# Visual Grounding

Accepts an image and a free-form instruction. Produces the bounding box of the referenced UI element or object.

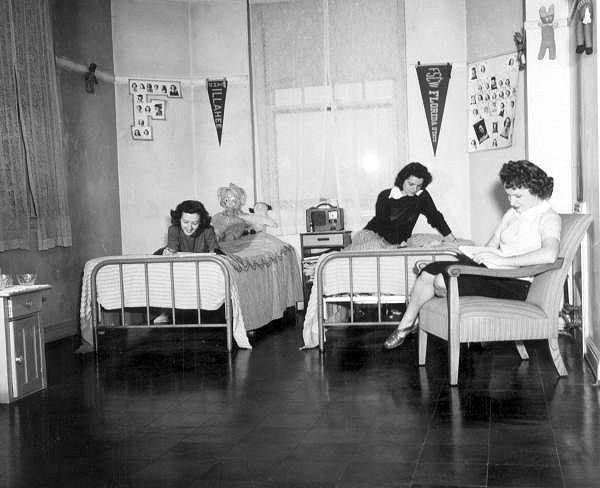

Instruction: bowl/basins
[0,272,38,292]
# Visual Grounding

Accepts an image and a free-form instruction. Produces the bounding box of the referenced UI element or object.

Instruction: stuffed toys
[210,183,245,241]
[243,202,277,235]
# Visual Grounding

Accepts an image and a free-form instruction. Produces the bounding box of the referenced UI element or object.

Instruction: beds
[72,232,299,354]
[299,231,477,354]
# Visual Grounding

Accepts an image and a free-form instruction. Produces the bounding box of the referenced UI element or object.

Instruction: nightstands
[300,230,353,306]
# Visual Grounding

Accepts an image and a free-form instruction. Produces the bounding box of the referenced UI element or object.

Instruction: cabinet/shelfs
[1,281,53,404]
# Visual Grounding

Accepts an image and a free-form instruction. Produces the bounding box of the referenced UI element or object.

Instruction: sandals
[384,315,420,351]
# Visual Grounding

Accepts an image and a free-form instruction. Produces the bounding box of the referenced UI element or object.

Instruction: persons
[170,85,179,96]
[477,125,488,143]
[162,201,220,253]
[571,0,593,54]
[346,163,456,251]
[383,159,561,350]
[537,5,556,61]
[501,118,510,138]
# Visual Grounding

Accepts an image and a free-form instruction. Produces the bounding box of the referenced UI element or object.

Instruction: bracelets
[453,238,456,243]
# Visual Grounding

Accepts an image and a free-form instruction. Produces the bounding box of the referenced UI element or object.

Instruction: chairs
[406,212,591,388]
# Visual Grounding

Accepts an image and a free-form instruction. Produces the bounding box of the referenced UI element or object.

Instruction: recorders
[305,202,344,232]
